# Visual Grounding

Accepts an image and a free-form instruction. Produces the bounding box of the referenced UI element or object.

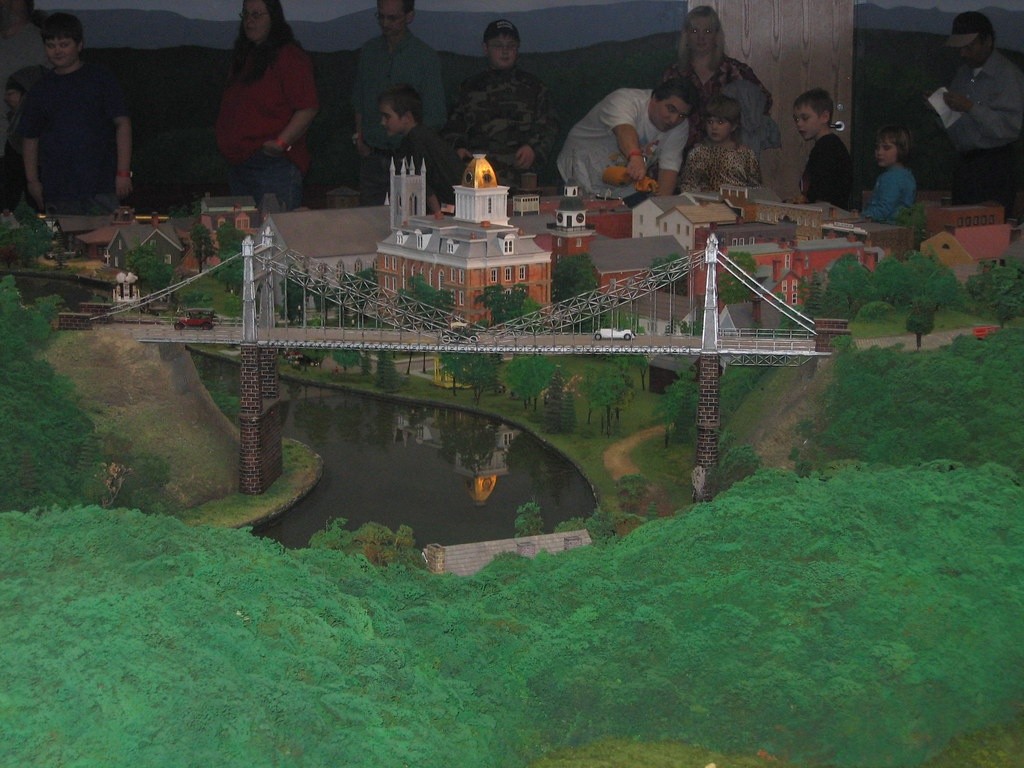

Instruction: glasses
[375,12,407,22]
[488,43,519,51]
[239,11,269,19]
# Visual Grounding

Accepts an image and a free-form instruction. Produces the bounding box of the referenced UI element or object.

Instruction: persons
[212,0,321,214]
[924,12,1024,224]
[0,0,153,216]
[352,0,915,224]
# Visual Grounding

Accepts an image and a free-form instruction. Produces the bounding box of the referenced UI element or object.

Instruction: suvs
[174,308,215,331]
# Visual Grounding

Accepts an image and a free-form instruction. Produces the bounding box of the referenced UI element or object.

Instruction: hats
[946,11,991,46]
[484,20,520,42]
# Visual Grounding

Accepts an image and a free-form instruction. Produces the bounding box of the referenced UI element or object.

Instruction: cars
[441,327,480,343]
[594,328,635,341]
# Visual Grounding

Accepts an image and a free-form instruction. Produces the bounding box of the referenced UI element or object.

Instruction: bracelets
[117,169,133,178]
[628,150,648,164]
[355,131,363,140]
[273,136,292,152]
